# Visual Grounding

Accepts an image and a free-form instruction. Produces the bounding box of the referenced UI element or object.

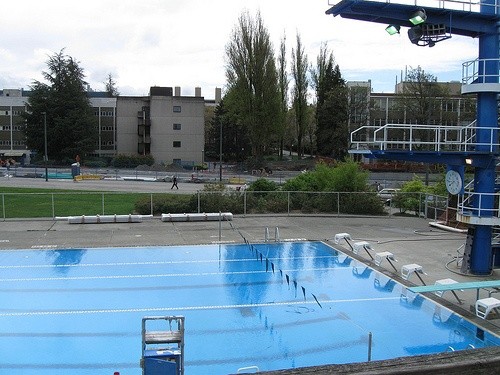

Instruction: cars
[422,195,447,215]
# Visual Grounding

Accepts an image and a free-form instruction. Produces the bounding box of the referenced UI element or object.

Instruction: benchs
[162,212,233,222]
[67,215,142,222]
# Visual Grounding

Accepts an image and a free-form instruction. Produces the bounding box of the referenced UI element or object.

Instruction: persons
[171,176,178,190]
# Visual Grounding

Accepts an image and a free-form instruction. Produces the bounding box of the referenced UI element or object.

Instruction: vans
[375,188,403,206]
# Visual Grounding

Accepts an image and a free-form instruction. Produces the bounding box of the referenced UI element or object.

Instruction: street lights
[39,112,49,182]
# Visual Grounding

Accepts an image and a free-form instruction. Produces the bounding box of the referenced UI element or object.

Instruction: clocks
[445,170,462,195]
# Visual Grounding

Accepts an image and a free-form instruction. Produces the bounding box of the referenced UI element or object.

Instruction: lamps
[385,24,400,35]
[427,37,436,47]
[407,27,423,45]
[465,157,473,164]
[408,8,427,25]
